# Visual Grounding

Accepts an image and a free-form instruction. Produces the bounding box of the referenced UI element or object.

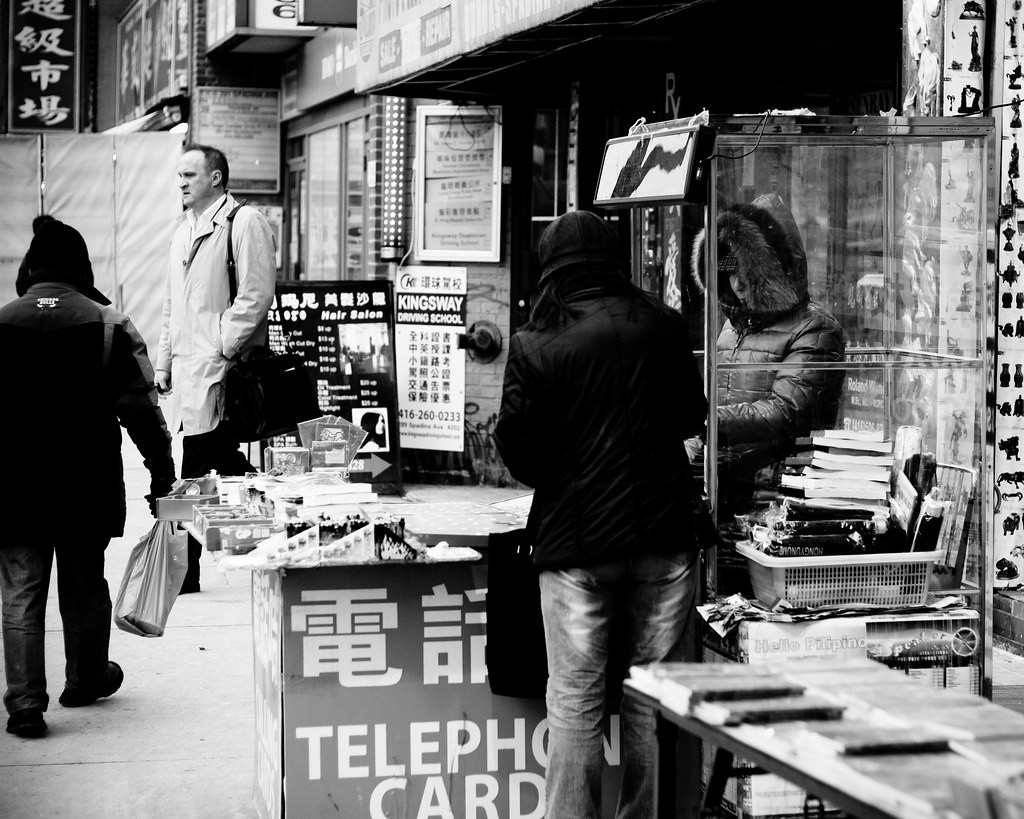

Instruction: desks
[623,678,930,818]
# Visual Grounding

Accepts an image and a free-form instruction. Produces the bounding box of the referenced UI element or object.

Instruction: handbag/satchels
[113,519,187,639]
[219,345,318,445]
[487,489,641,714]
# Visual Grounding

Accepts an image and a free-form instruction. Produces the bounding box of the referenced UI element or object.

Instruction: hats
[16,214,112,307]
[537,209,624,286]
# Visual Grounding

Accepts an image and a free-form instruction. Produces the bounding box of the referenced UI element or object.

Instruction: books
[764,429,895,553]
[629,654,1022,819]
[293,481,383,519]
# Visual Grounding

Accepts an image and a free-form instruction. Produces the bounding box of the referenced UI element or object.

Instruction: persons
[490,209,722,819]
[684,190,849,819]
[153,144,277,596]
[0,212,180,738]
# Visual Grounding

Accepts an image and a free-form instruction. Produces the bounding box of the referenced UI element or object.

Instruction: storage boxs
[193,505,273,552]
[156,494,220,520]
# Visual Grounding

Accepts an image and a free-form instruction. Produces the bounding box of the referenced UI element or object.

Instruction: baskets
[735,539,947,609]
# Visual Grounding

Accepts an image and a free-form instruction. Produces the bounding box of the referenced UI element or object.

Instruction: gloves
[142,480,174,519]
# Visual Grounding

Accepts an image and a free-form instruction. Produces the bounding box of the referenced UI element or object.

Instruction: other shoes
[6,714,51,738]
[59,661,124,708]
